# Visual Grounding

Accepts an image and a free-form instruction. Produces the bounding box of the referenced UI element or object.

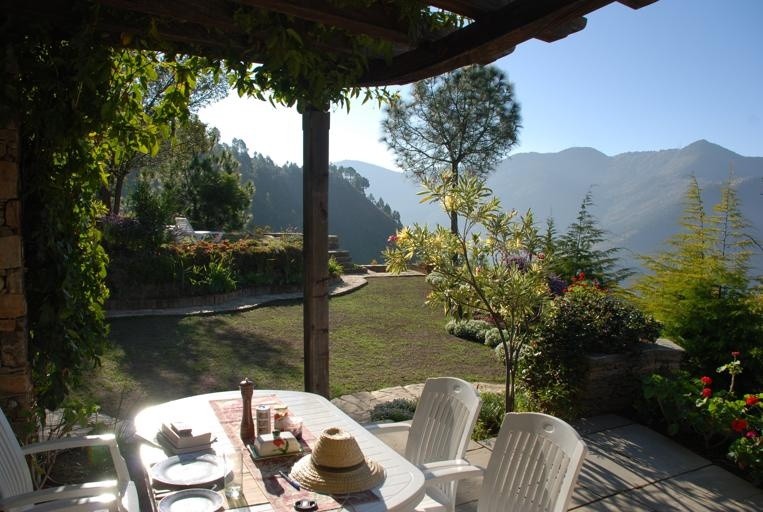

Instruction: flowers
[694,350,763,473]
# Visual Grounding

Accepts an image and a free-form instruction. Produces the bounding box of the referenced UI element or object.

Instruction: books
[153,422,211,455]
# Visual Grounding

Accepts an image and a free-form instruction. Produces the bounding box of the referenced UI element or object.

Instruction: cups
[223,449,244,498]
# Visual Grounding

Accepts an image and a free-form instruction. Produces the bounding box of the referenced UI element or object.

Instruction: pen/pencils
[279,470,299,489]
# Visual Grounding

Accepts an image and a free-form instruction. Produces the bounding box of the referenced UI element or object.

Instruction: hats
[290,427,387,495]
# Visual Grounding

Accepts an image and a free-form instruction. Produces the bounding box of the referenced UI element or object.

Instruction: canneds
[256,405,270,435]
[274,405,288,431]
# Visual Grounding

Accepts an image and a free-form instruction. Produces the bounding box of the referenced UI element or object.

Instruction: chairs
[362,375,588,512]
[0,408,141,511]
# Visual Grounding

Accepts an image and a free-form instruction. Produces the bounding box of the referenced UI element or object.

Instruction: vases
[707,430,740,475]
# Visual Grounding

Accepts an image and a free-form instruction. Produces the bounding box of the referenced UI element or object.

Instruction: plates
[159,488,224,512]
[247,438,311,460]
[150,452,233,486]
